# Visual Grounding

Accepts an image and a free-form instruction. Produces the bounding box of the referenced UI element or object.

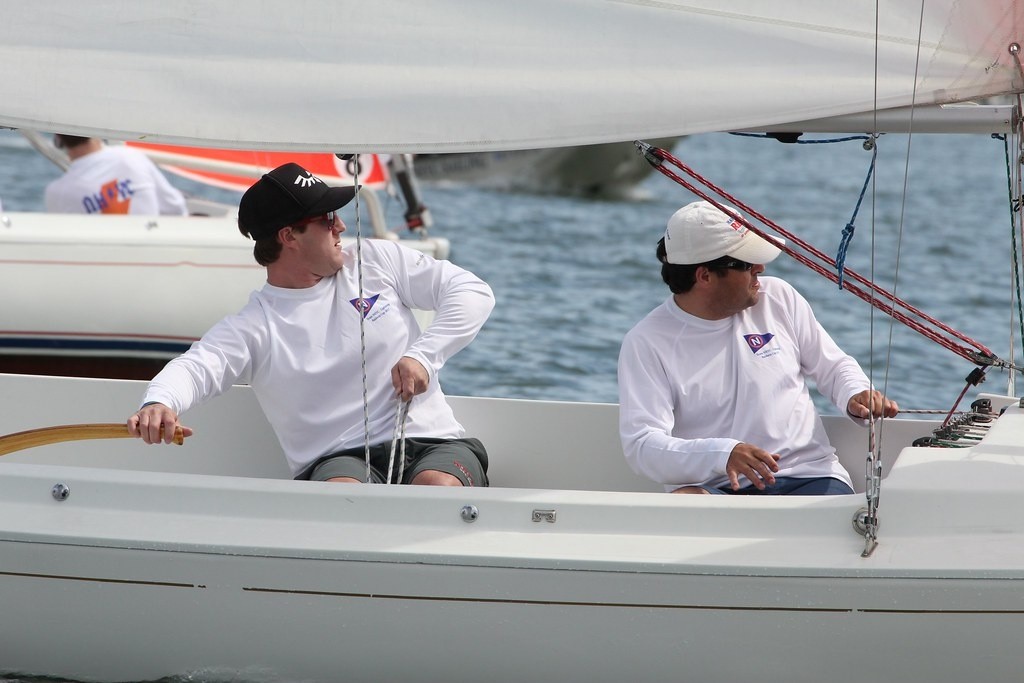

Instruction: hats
[238,163,362,240]
[661,200,785,264]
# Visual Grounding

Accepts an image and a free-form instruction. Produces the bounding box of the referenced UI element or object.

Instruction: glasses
[706,259,753,270]
[286,211,335,227]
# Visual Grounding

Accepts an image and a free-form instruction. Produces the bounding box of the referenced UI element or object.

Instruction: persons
[46,133,187,216]
[618,200,898,496]
[126,162,496,486]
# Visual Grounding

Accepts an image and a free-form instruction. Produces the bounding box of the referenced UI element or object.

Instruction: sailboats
[0,123,453,381]
[0,0,1024,683]
